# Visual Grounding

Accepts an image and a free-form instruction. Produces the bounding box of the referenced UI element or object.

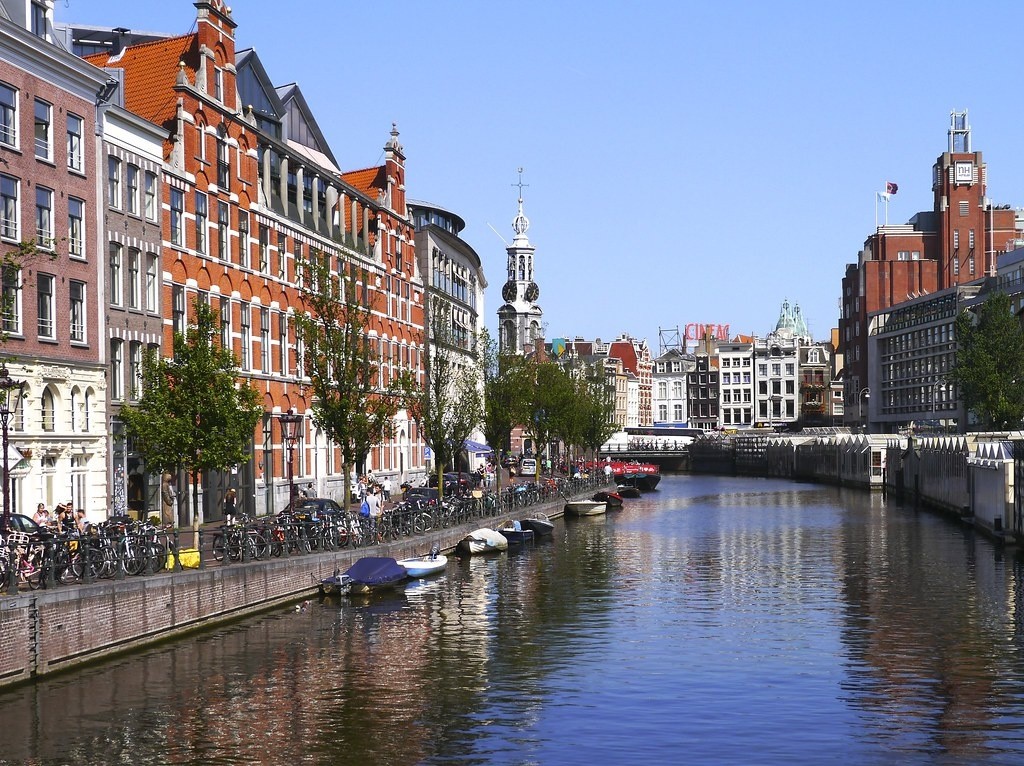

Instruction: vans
[518,458,537,476]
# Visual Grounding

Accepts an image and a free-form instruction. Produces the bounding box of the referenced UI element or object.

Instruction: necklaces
[37,511,42,517]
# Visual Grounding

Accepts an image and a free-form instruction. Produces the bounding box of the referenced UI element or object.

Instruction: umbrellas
[914,419,942,434]
[444,438,495,457]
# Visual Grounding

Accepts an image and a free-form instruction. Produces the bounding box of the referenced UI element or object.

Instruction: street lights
[932,379,948,421]
[278,410,304,511]
[858,386,871,433]
[0,369,25,544]
[639,398,651,426]
[551,440,559,477]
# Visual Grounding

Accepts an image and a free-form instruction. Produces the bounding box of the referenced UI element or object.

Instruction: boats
[454,527,509,556]
[617,485,642,498]
[560,491,608,517]
[495,518,534,544]
[395,542,449,579]
[592,491,623,507]
[318,553,408,597]
[519,512,554,535]
[611,459,662,491]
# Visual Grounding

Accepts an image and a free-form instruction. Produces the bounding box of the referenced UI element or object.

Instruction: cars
[501,456,517,467]
[278,495,344,523]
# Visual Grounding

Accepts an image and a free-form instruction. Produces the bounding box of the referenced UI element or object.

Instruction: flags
[877,181,898,203]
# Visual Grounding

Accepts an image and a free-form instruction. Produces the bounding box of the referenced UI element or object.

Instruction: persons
[373,484,384,540]
[224,488,239,526]
[476,448,613,518]
[360,488,381,546]
[32,502,88,560]
[358,469,392,504]
[377,190,387,207]
[293,481,316,500]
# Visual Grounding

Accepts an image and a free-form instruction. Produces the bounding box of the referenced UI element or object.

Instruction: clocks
[502,281,518,303]
[527,283,540,301]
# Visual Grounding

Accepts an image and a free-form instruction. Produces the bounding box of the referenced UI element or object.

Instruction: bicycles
[210,464,606,564]
[0,509,178,592]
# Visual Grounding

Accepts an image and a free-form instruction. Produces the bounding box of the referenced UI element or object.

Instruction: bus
[622,425,717,451]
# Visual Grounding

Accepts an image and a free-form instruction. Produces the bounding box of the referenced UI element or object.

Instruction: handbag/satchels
[360,497,370,518]
